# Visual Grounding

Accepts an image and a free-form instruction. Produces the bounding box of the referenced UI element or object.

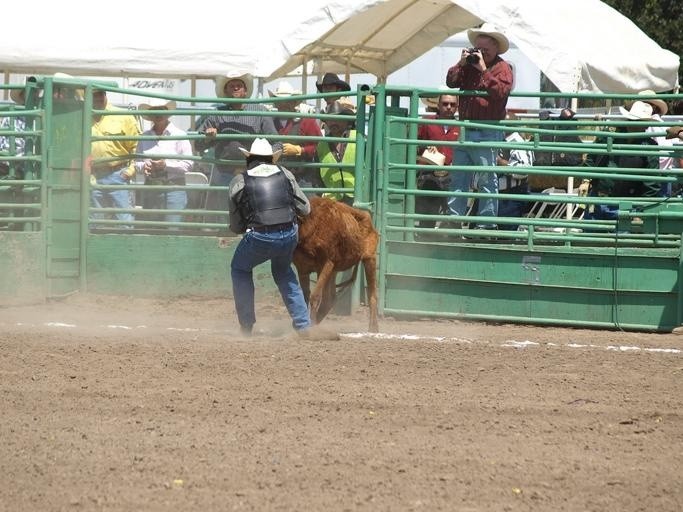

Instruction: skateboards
[291,196,379,333]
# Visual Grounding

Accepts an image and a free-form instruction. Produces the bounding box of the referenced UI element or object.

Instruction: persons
[89,88,139,228]
[440,26,513,244]
[265,83,322,183]
[416,93,535,240]
[226,136,310,338]
[314,74,359,204]
[133,100,194,230]
[193,77,284,236]
[578,90,681,233]
[1,88,42,179]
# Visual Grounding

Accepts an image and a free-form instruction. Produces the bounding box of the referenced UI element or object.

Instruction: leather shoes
[666,126,682,139]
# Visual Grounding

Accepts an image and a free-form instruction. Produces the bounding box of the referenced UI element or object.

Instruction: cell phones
[465,48,482,65]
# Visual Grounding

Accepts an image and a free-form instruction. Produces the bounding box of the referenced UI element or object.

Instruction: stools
[465,127,482,132]
[95,164,128,176]
[257,225,294,232]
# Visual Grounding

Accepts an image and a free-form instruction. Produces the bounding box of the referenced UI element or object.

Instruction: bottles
[638,89,669,116]
[319,100,356,124]
[421,96,441,108]
[215,71,254,98]
[267,82,302,105]
[137,95,176,119]
[619,101,665,121]
[9,88,26,104]
[415,149,445,166]
[315,73,352,92]
[466,22,509,54]
[239,137,283,163]
